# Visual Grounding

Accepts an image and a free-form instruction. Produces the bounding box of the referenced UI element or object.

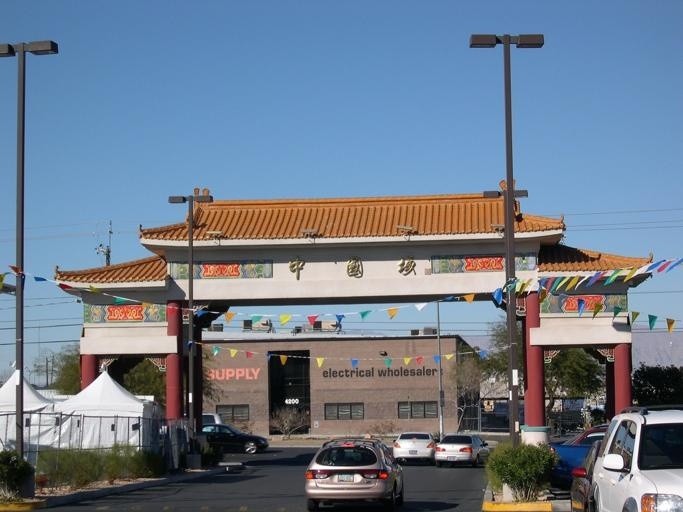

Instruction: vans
[159,414,237,435]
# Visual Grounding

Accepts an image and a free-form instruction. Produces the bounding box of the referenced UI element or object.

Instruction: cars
[435,434,490,467]
[304,439,404,511]
[547,409,683,512]
[393,432,435,465]
[201,426,268,453]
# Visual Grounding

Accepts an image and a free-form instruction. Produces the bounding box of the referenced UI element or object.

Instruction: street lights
[470,36,544,449]
[168,196,211,470]
[1,41,58,499]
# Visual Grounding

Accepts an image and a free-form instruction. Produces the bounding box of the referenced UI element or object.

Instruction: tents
[0,369,156,457]
